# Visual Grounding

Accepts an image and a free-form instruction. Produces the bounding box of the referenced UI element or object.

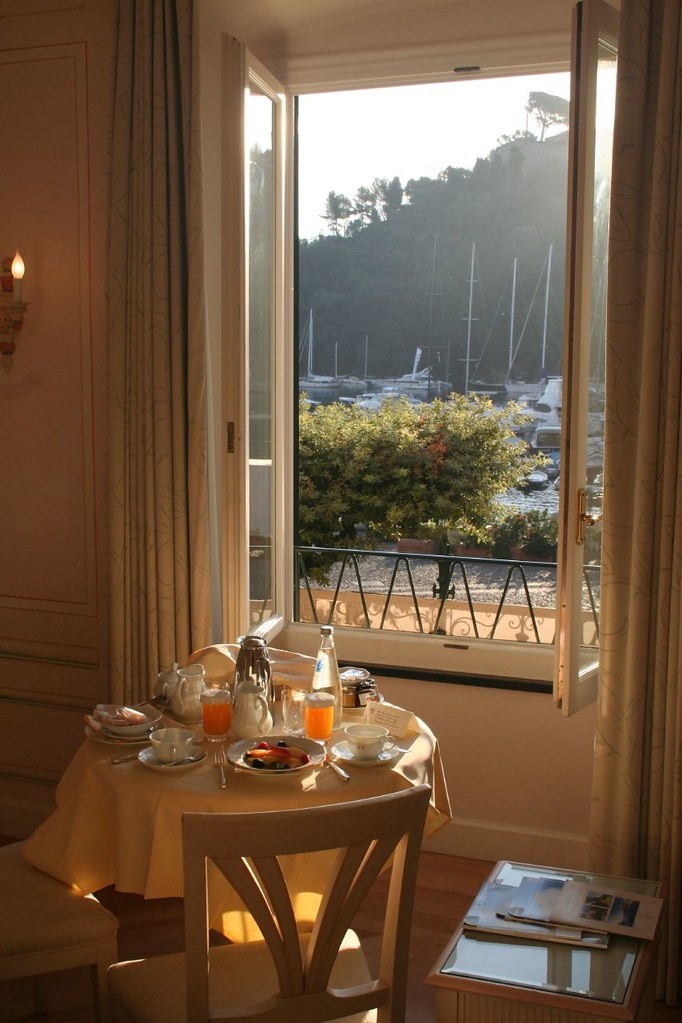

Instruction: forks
[213,746,229,789]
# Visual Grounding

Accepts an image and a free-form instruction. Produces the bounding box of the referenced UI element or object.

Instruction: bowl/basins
[102,705,163,736]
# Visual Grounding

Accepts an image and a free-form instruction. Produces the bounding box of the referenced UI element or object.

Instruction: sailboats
[303,237,561,497]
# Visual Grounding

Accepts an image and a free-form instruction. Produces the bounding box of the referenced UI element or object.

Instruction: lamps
[0,245,31,372]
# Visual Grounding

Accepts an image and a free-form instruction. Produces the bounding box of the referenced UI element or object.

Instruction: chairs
[105,785,433,1023]
[0,839,120,1022]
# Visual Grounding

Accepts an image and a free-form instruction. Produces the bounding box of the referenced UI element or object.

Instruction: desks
[424,861,664,1023]
[20,696,452,944]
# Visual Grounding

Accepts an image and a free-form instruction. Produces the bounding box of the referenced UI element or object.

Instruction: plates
[226,734,327,776]
[331,740,400,766]
[137,746,208,774]
[84,721,167,745]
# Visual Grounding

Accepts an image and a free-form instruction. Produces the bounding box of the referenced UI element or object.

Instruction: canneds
[341,678,380,709]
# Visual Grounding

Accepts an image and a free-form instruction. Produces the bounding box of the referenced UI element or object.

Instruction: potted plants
[399,499,558,561]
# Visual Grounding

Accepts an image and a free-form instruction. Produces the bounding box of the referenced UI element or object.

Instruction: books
[463,876,663,950]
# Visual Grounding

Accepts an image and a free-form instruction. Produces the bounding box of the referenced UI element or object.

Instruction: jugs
[170,663,208,724]
[154,663,182,706]
[229,682,273,738]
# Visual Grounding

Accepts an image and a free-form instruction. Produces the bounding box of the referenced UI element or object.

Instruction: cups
[149,728,195,763]
[280,688,308,736]
[343,724,396,759]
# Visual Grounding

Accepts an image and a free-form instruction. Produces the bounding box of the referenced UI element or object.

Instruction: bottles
[310,625,343,730]
[305,692,336,741]
[200,688,233,743]
